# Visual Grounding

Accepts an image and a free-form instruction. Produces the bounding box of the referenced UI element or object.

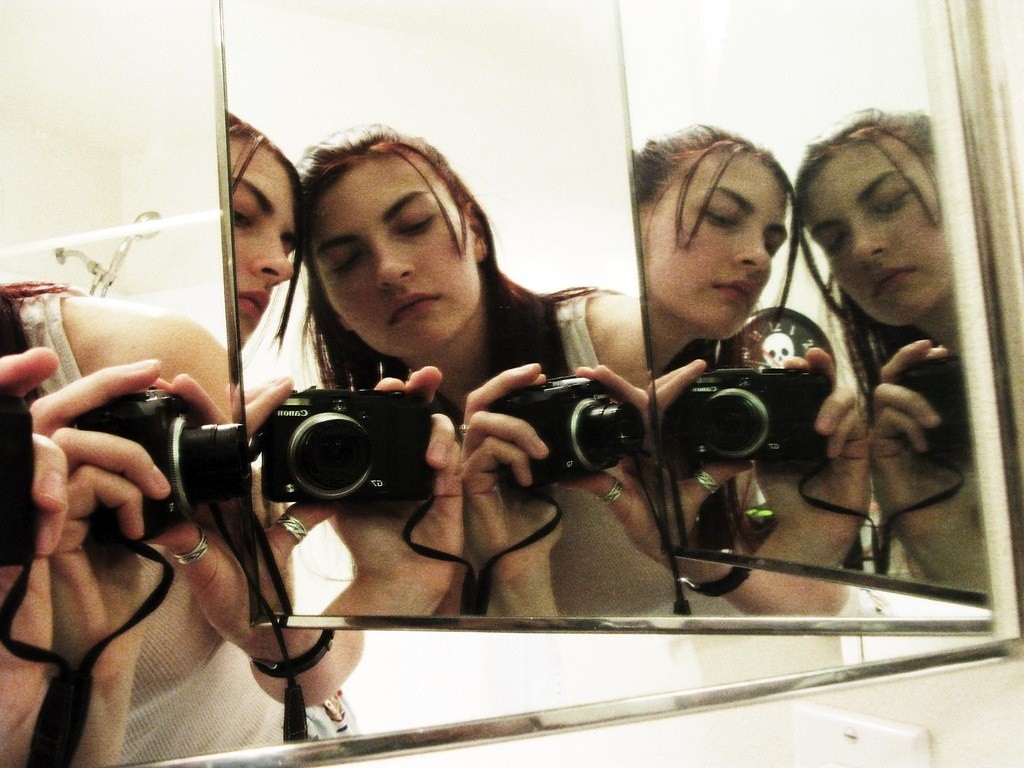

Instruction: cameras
[71,391,253,557]
[666,366,832,465]
[0,394,38,566]
[262,392,438,510]
[488,375,645,499]
[897,356,973,457]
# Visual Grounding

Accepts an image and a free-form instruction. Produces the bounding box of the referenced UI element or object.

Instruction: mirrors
[208,0,1007,636]
[122,44,1021,768]
[616,0,997,609]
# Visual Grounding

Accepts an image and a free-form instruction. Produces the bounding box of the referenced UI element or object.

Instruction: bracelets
[678,567,751,598]
[249,629,334,679]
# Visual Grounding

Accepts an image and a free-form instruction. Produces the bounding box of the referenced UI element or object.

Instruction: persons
[0,282,364,768]
[228,112,850,615]
[631,113,990,592]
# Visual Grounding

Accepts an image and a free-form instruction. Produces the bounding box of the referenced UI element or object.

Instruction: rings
[694,468,717,493]
[599,477,624,503]
[169,524,210,565]
[278,514,308,544]
